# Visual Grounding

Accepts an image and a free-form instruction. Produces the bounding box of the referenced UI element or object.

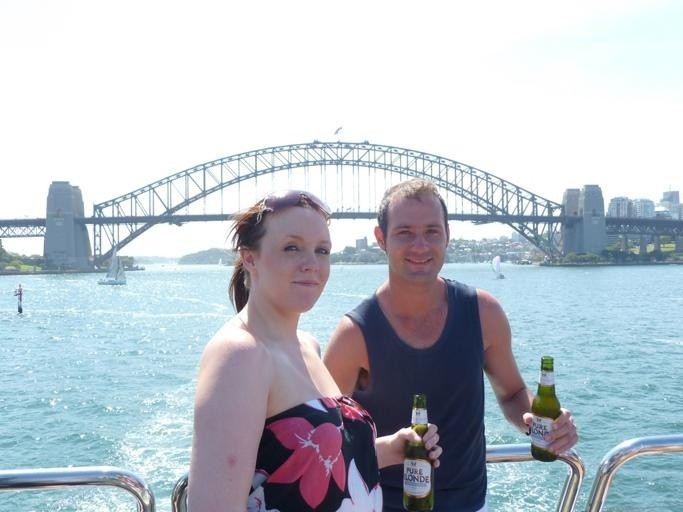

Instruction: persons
[187,190,444,512]
[323,179,579,511]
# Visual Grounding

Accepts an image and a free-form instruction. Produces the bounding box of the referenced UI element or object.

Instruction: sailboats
[97,246,127,285]
[492,255,505,279]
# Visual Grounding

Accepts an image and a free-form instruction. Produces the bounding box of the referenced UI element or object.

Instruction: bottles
[402,392,434,511]
[531,355,561,462]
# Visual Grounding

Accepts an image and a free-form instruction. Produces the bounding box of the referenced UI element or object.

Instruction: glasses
[264,188,332,215]
